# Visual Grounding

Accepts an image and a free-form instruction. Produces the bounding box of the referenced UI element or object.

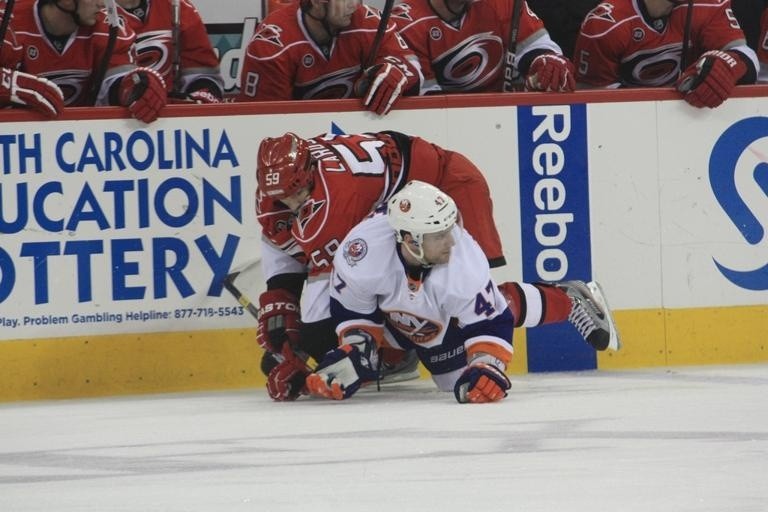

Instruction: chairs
[260,351,285,377]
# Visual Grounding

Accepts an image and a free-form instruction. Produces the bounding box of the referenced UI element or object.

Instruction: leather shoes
[522,51,576,94]
[305,343,368,400]
[351,54,420,116]
[674,49,750,109]
[117,68,167,125]
[265,352,319,403]
[452,353,511,403]
[254,289,300,356]
[0,67,65,119]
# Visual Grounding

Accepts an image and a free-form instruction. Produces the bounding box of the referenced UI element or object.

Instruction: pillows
[387,179,457,244]
[256,132,317,203]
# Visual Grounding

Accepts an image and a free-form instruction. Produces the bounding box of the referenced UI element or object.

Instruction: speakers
[532,284,610,353]
[368,347,419,379]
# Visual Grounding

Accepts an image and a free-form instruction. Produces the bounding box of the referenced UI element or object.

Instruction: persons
[573,1,767,108]
[1,0,225,121]
[262,178,515,405]
[227,1,576,116]
[253,129,610,400]
